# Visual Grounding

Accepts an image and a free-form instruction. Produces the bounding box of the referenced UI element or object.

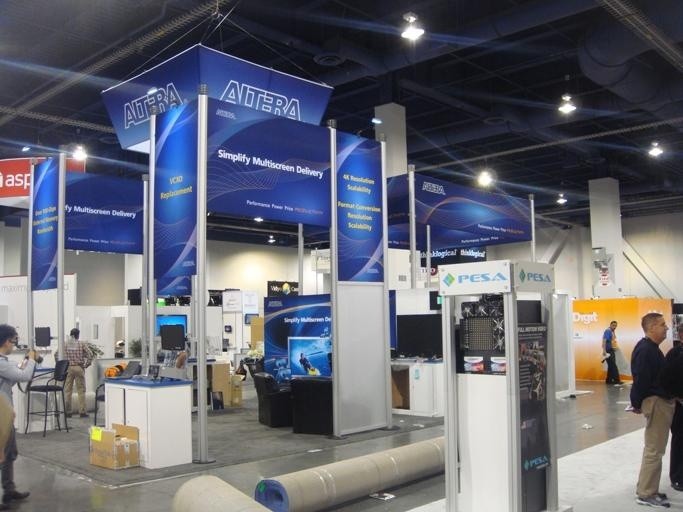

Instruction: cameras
[25,349,43,364]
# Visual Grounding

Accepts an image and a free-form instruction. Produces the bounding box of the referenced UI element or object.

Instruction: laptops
[107,361,140,380]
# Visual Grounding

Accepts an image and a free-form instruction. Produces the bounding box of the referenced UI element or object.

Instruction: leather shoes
[671,482,683,491]
[2,492,30,503]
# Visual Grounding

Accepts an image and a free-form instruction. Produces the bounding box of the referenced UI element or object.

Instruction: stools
[92,361,141,425]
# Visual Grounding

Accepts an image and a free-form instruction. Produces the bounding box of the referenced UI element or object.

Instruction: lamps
[591,245,610,273]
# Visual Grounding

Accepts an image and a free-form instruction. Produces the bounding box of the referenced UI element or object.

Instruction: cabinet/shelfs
[389,359,444,418]
[189,363,229,412]
[101,377,195,471]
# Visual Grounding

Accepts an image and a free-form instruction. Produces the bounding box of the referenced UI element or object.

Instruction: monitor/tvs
[160,324,185,350]
[35,327,50,346]
[156,314,187,336]
[245,314,259,324]
[287,336,332,377]
[397,314,442,362]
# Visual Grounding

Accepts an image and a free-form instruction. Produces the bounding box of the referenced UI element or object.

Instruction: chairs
[290,378,332,434]
[252,371,293,428]
[24,360,73,436]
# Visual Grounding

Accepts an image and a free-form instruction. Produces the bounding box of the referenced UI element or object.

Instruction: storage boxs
[230,373,245,406]
[89,421,141,470]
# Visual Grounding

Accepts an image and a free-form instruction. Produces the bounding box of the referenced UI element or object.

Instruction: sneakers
[635,492,670,509]
[80,413,89,417]
[67,414,72,418]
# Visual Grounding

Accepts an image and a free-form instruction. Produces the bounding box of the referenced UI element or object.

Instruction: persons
[299,353,315,374]
[631,312,676,509]
[602,321,624,385]
[0,325,39,508]
[666,322,683,490]
[55,328,93,417]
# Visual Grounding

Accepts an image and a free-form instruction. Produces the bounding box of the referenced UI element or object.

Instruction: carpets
[6,378,444,488]
